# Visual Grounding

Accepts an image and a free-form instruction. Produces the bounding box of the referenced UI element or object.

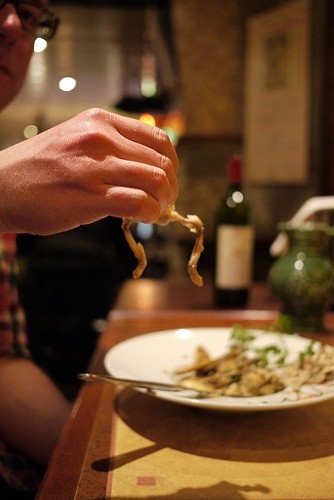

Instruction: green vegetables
[228,324,314,370]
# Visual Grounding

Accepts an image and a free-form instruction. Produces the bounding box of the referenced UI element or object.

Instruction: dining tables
[35,277,334,500]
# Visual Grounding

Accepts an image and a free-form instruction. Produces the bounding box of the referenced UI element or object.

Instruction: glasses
[0,0,60,40]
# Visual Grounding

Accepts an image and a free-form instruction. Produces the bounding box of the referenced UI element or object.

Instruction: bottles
[209,157,253,311]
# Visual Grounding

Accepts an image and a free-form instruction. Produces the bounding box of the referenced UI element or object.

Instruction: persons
[0,0,178,500]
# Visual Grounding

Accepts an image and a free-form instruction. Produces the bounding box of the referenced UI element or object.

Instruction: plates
[104,328,334,414]
